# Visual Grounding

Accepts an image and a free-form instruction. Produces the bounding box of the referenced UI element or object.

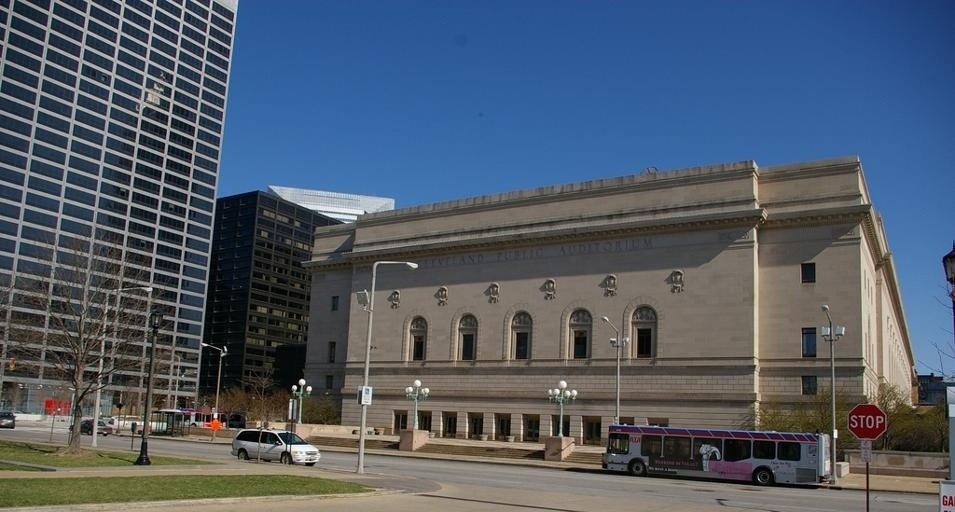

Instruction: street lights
[355,259,419,473]
[200,341,229,420]
[547,379,578,436]
[942,240,955,344]
[600,315,630,425]
[405,378,431,430]
[291,378,313,423]
[133,304,164,465]
[817,303,847,483]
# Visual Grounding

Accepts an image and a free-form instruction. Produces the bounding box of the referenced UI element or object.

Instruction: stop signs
[847,403,887,441]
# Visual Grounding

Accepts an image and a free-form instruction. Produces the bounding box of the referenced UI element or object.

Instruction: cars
[68,419,112,436]
[105,409,246,434]
[0,411,16,428]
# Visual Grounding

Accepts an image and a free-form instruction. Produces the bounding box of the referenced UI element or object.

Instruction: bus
[602,423,831,487]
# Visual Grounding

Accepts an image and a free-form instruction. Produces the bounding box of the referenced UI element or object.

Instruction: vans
[231,428,321,467]
